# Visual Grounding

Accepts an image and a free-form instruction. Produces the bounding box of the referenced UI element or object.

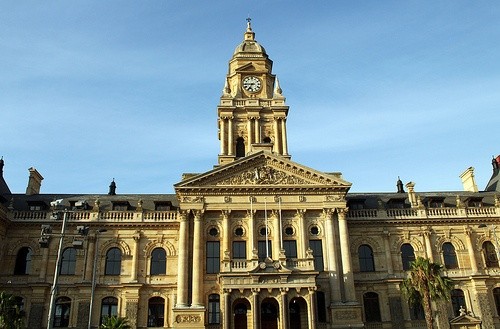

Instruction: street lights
[37,197,107,329]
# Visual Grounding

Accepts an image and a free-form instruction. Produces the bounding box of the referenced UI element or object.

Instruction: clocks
[243,74,262,95]
